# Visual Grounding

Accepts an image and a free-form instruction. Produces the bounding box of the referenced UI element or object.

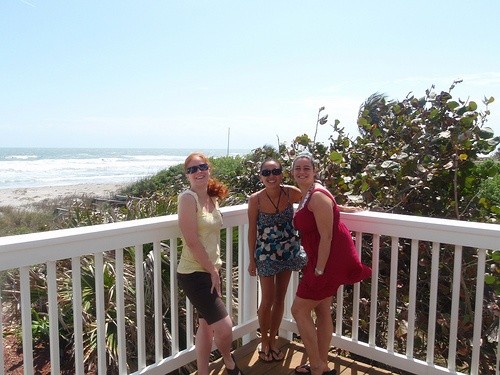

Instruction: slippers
[258,346,273,362]
[270,347,284,361]
[295,363,336,375]
[222,353,243,375]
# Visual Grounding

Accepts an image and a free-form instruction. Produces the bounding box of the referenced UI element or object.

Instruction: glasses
[261,169,283,177]
[185,164,209,174]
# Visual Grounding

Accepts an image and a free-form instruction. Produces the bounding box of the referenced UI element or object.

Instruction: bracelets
[315,269,322,275]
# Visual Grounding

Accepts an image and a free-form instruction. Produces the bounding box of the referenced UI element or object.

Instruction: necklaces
[264,190,282,213]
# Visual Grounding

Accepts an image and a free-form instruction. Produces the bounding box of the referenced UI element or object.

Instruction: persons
[177,152,243,375]
[290,153,372,375]
[248,160,363,362]
